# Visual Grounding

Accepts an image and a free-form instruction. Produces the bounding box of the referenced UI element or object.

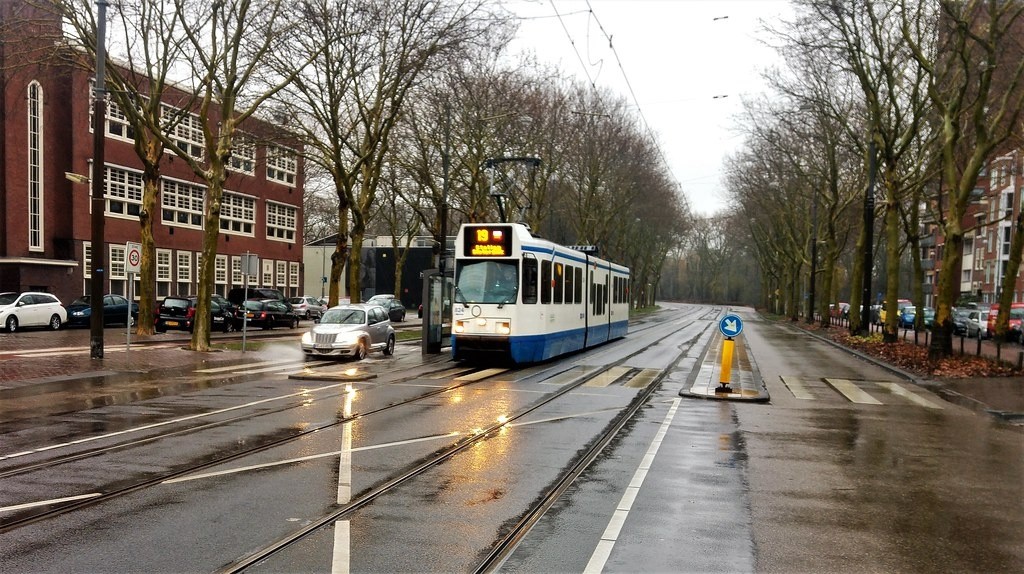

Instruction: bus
[450,156,629,367]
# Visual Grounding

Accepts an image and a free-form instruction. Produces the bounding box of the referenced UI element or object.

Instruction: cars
[828,299,934,330]
[951,301,1024,345]
[302,303,396,361]
[66,294,139,328]
[0,292,69,333]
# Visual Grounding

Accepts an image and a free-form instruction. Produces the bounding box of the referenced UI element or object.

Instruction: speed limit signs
[129,250,139,266]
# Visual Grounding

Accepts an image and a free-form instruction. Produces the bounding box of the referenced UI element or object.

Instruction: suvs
[154,287,406,334]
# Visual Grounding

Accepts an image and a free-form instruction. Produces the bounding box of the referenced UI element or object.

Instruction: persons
[496,269,517,291]
